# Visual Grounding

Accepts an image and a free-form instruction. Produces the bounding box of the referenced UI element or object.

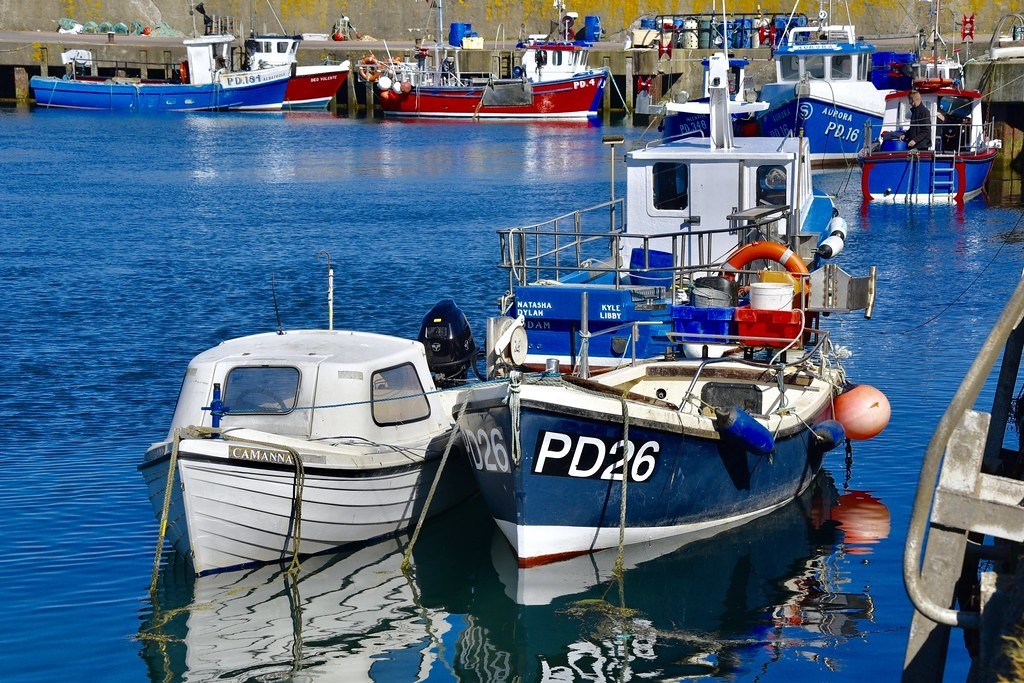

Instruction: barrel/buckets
[749,282,795,312]
[630,248,678,291]
[585,16,601,42]
[880,139,907,153]
[449,23,471,46]
[692,288,732,308]
[642,11,811,49]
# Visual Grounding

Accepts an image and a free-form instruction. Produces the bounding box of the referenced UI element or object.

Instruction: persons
[899,90,930,150]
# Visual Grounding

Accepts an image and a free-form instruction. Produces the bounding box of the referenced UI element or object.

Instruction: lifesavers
[360,57,381,80]
[720,240,810,299]
[180,60,189,83]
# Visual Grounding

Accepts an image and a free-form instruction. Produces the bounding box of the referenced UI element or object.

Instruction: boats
[856,0,1004,210]
[648,0,886,173]
[22,0,304,116]
[355,0,615,121]
[446,0,883,573]
[136,327,513,580]
[179,0,351,113]
